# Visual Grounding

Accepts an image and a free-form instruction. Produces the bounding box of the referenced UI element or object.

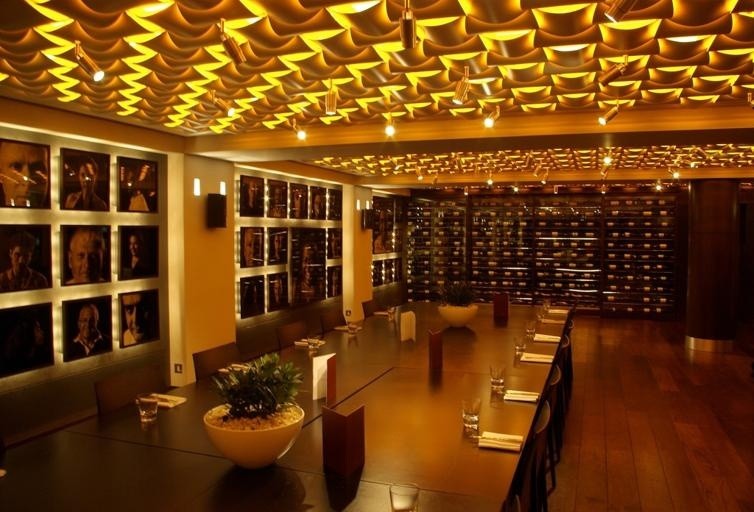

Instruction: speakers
[207,193,227,228]
[362,208,381,229]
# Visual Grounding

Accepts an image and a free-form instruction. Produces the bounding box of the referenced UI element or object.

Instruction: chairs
[192,341,237,382]
[276,319,307,351]
[361,299,380,320]
[1,298,573,512]
[321,311,338,333]
[504,311,574,511]
[91,364,160,413]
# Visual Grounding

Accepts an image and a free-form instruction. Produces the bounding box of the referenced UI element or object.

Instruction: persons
[242,178,340,314]
[373,209,391,254]
[0,141,159,361]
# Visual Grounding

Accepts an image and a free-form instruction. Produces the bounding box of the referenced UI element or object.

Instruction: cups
[389,483,420,512]
[461,397,481,428]
[525,320,536,336]
[307,349,319,361]
[137,396,159,421]
[307,335,320,348]
[514,337,526,352]
[489,364,507,389]
[462,429,479,448]
[490,390,505,410]
[387,307,398,319]
[514,353,523,368]
[347,319,359,333]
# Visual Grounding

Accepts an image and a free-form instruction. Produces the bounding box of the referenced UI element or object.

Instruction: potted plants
[200,351,305,469]
[436,281,480,328]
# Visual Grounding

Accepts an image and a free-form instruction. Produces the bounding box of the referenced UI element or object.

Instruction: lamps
[604,1,634,24]
[414,148,696,196]
[598,54,628,88]
[385,113,396,136]
[75,41,105,81]
[323,78,338,116]
[451,65,469,106]
[598,98,620,125]
[399,1,416,49]
[484,106,501,127]
[210,90,235,117]
[221,19,246,67]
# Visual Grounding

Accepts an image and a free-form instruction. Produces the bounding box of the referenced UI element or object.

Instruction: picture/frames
[239,175,343,319]
[0,135,161,378]
